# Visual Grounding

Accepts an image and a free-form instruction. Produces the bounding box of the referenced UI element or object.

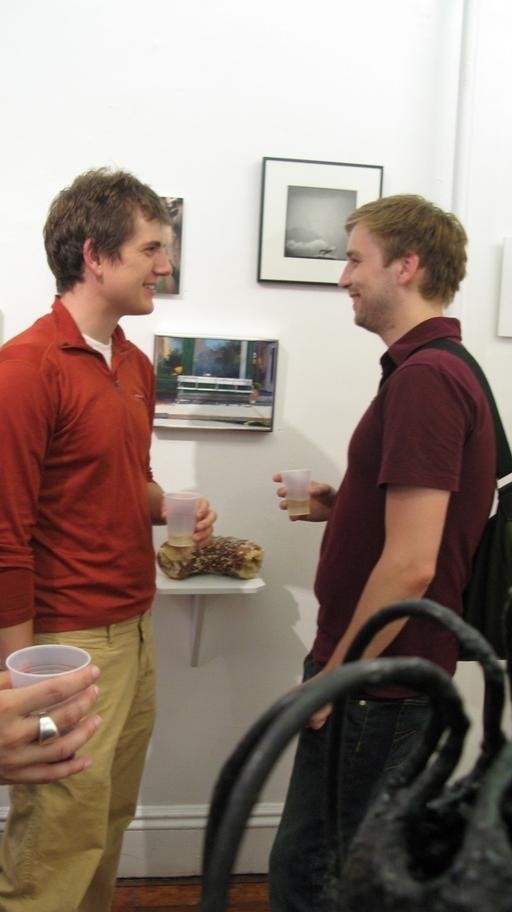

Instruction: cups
[279,469,314,517]
[7,644,90,689]
[162,491,200,548]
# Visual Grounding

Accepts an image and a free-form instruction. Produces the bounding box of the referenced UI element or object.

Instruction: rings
[38,709,60,746]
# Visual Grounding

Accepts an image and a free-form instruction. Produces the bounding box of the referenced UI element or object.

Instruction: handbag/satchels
[457,482,512,661]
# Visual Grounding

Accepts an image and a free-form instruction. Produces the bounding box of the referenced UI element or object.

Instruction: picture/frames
[258,157,383,284]
[153,335,278,432]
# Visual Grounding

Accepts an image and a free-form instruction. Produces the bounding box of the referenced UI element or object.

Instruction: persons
[0,166,219,912]
[0,660,102,786]
[269,195,498,911]
